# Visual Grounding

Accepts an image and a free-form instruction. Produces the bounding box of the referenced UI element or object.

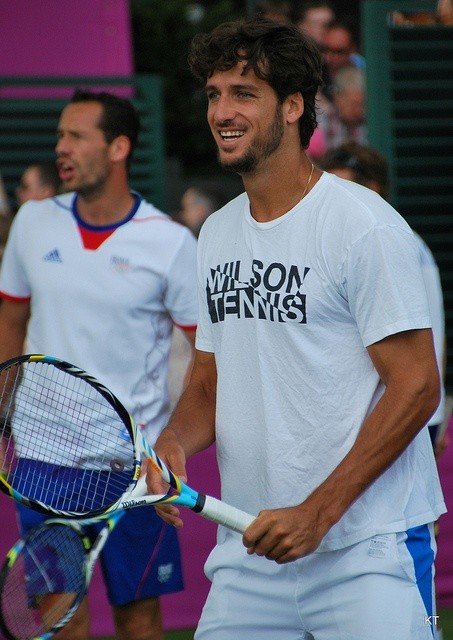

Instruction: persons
[300,8,332,46]
[321,143,389,195]
[309,65,366,155]
[12,164,58,203]
[318,25,358,101]
[177,184,212,235]
[147,22,452,639]
[1,88,198,640]
